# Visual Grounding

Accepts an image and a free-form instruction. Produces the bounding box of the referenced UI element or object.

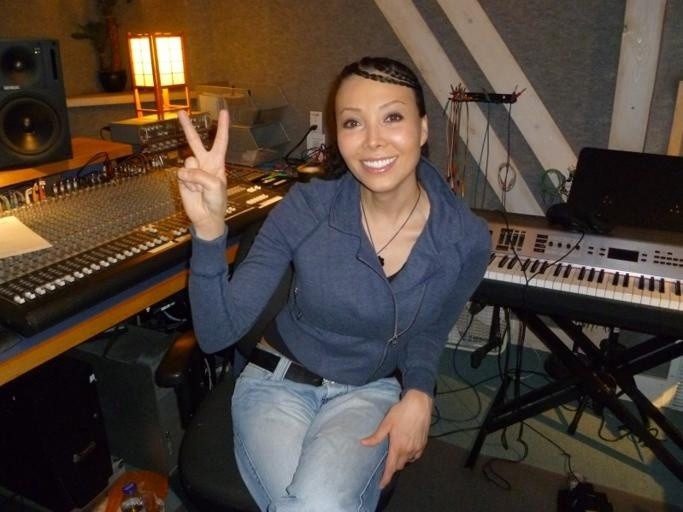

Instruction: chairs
[156,220,436,511]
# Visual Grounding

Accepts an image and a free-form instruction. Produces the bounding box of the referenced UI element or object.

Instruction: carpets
[386,436,681,511]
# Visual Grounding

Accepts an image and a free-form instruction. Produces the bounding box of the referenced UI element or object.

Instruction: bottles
[121,481,145,512]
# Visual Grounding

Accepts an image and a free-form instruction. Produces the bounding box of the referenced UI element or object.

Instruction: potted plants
[72,0,126,92]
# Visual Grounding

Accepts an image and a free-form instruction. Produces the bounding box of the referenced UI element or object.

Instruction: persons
[176,57,491,512]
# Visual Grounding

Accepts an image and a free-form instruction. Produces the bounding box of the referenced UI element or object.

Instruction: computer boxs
[0,353,114,511]
[64,326,185,483]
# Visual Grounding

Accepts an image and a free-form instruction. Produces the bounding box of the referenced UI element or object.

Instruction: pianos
[468,206,683,337]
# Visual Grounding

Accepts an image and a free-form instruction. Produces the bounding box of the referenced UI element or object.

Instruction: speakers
[1,36,73,172]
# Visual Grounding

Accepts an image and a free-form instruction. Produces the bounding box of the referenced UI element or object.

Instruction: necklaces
[360,184,421,266]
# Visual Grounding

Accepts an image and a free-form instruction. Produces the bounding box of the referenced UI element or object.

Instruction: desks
[0,136,314,386]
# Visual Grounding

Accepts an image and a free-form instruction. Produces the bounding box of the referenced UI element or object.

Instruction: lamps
[126,31,191,121]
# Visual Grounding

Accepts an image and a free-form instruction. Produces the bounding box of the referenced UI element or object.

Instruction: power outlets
[309,111,322,134]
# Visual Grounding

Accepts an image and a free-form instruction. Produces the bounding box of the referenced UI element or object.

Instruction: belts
[250,347,323,386]
